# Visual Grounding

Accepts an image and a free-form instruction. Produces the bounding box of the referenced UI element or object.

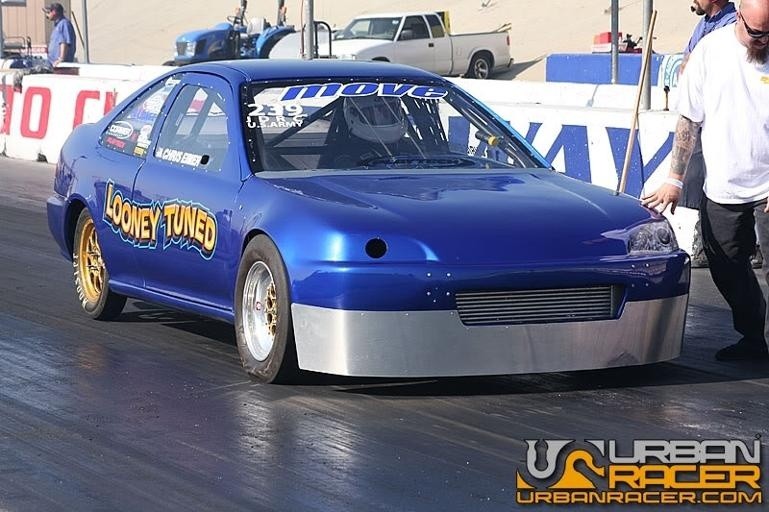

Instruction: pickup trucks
[314,10,515,80]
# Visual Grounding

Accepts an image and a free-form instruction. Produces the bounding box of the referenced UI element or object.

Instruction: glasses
[740,13,769,39]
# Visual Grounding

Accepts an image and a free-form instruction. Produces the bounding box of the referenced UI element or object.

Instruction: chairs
[373,24,426,38]
[318,95,430,170]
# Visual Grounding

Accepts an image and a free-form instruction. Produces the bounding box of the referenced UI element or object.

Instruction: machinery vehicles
[163,13,295,65]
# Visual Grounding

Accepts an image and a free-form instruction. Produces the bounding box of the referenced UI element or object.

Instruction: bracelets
[663,179,684,190]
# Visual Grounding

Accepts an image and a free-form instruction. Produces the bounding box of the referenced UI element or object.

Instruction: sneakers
[716,334,769,362]
[751,245,764,270]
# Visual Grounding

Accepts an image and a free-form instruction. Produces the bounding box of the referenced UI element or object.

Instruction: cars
[45,57,694,389]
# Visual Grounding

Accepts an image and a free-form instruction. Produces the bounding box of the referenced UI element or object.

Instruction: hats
[41,3,63,12]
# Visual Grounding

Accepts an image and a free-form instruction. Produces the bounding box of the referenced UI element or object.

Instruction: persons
[38,2,78,68]
[641,1,769,361]
[677,0,765,270]
